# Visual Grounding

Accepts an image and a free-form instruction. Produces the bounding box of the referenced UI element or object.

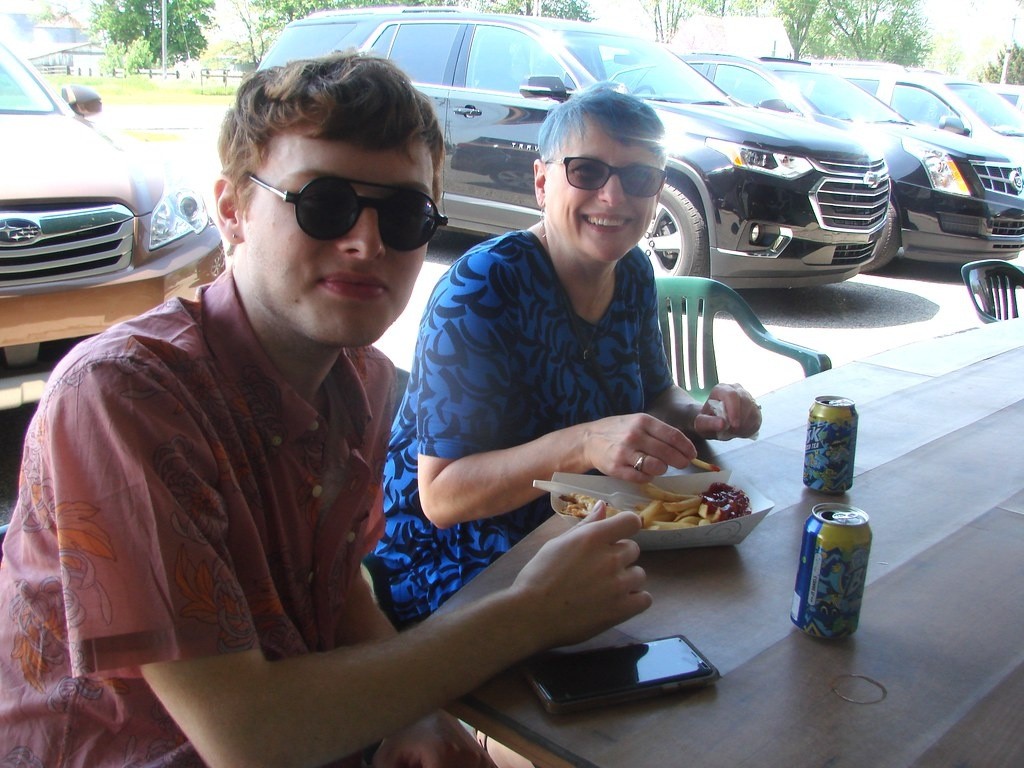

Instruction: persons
[0,52,653,768]
[365,87,762,631]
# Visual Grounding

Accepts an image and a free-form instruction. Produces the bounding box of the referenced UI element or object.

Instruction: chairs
[530,44,604,91]
[471,39,522,94]
[961,259,1024,324]
[654,275,832,407]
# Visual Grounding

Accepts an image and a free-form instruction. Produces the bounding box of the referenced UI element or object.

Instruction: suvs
[257,5,890,313]
[0,33,230,438]
[675,51,1024,268]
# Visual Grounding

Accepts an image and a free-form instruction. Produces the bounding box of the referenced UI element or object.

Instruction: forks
[533,478,651,513]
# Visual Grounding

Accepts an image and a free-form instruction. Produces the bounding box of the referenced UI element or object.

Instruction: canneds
[802,395,858,493]
[789,503,873,640]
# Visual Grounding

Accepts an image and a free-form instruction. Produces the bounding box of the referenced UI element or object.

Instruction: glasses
[547,156,668,198]
[247,172,448,252]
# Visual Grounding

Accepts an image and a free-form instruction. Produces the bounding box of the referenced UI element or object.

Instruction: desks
[442,315,1024,768]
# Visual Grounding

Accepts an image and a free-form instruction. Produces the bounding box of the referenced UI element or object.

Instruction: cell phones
[521,635,721,716]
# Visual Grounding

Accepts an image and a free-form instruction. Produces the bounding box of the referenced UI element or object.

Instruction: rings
[758,405,762,409]
[633,455,647,471]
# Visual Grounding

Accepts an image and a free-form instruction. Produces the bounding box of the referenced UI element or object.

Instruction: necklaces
[556,275,618,359]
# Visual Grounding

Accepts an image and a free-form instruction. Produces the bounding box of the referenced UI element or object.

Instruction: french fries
[632,456,734,531]
